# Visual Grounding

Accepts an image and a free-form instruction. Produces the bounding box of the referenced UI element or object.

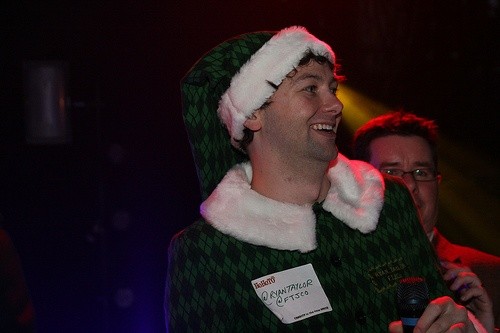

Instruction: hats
[182,26,335,203]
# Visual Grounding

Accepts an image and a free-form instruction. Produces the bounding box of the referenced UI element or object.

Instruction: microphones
[397,277,428,333]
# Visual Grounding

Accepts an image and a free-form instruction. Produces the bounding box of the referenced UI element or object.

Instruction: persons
[349,112,500,333]
[165,25,487,333]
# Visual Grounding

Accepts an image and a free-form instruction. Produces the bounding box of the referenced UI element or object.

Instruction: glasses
[379,169,441,182]
